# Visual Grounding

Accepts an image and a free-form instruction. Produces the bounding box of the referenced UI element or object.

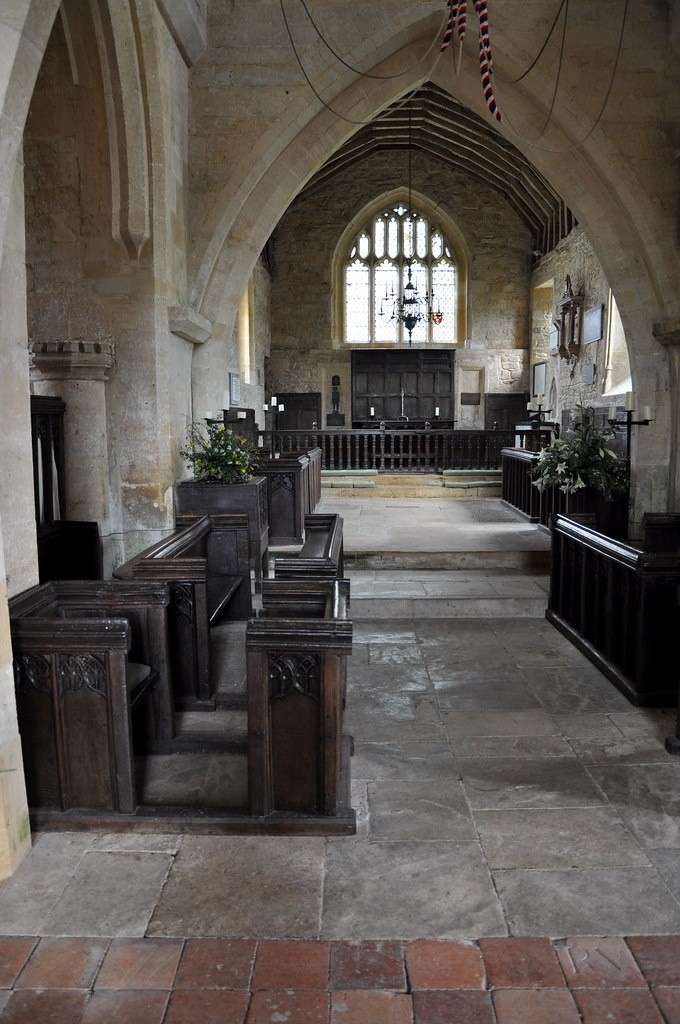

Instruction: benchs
[249,441,321,546]
[545,512,680,706]
[7,579,175,833]
[275,513,344,581]
[500,445,604,537]
[112,514,254,711]
[244,579,358,838]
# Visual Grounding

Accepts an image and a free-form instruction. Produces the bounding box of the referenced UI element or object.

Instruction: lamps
[379,93,442,347]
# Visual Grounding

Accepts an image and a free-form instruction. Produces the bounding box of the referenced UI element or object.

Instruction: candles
[262,404,268,410]
[370,407,374,415]
[435,406,439,416]
[527,402,532,410]
[538,393,543,404]
[278,403,284,411]
[271,396,277,406]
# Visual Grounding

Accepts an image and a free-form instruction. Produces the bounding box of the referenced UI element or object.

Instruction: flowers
[178,414,269,483]
[524,413,628,504]
